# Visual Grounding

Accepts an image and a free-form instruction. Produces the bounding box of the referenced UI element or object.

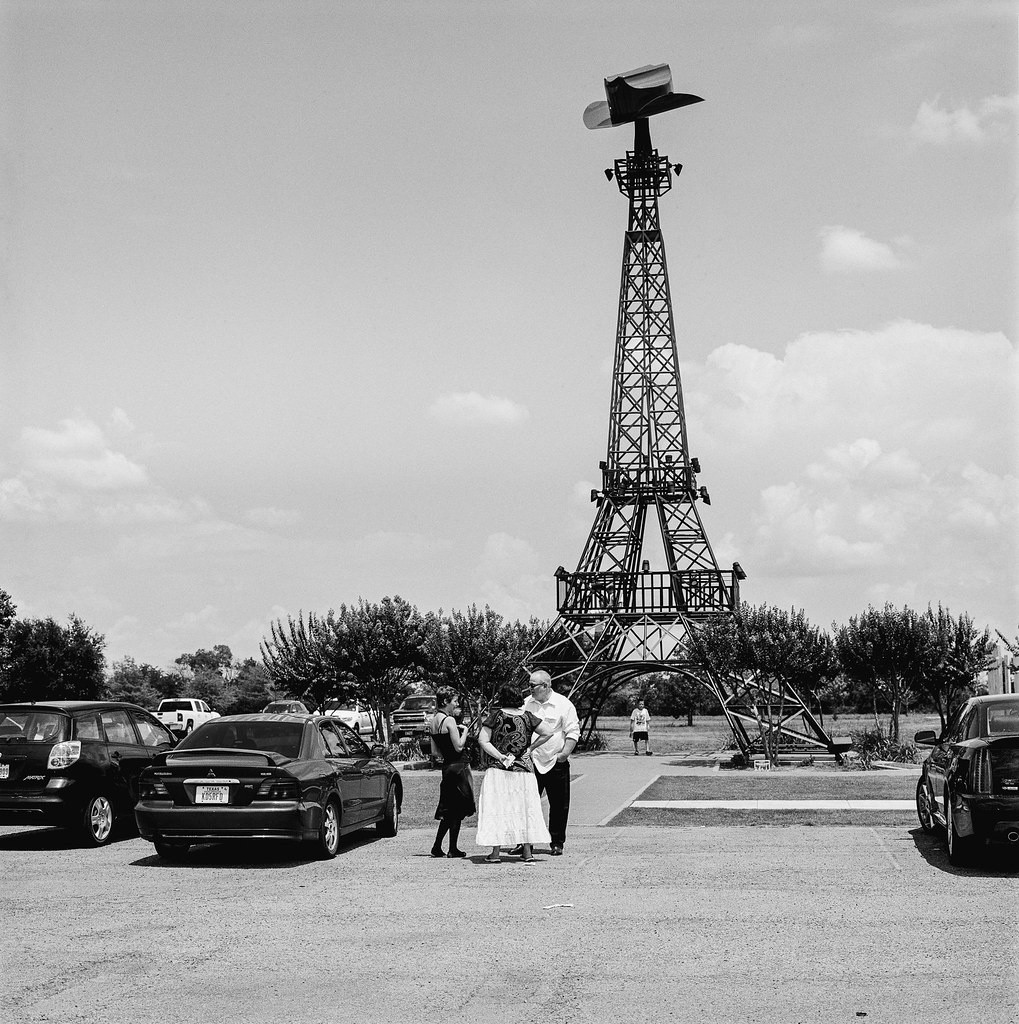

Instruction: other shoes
[646,751,652,756]
[431,846,446,857]
[634,751,639,755]
[447,848,466,858]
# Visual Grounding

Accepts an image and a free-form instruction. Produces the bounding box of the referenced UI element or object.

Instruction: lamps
[604,168,615,181]
[642,560,649,572]
[591,454,711,508]
[554,566,569,580]
[669,163,683,176]
[733,562,747,580]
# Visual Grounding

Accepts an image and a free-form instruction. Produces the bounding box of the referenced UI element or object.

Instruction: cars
[392,695,463,732]
[258,699,312,715]
[312,697,384,736]
[912,693,1017,870]
[130,712,404,859]
[0,700,182,846]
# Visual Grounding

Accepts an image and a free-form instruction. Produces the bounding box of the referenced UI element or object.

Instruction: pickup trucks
[146,697,222,741]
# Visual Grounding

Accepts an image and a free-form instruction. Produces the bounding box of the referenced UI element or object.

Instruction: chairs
[76,724,97,738]
[104,722,132,743]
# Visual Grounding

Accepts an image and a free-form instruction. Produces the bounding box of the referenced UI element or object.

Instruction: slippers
[520,854,535,862]
[485,854,501,863]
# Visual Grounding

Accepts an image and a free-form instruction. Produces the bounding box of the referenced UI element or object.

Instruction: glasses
[639,704,644,705]
[527,682,544,688]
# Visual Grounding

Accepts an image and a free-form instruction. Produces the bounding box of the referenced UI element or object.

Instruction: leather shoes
[550,844,562,856]
[508,845,523,855]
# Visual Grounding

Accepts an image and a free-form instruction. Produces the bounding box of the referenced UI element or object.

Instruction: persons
[475,680,554,863]
[508,670,581,855]
[630,698,652,755]
[429,685,476,857]
